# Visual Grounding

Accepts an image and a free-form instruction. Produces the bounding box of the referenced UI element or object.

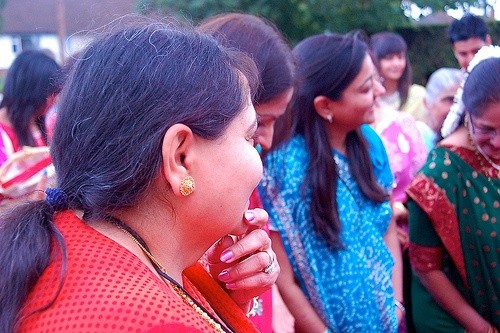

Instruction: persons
[416,68,464,154]
[257,33,405,333]
[403,44,500,333]
[370,32,425,121]
[0,24,280,333]
[450,15,492,73]
[197,13,296,333]
[367,66,429,333]
[0,49,66,215]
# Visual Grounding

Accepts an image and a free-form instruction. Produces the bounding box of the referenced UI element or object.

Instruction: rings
[261,250,275,274]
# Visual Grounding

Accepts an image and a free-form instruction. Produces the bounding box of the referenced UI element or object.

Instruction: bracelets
[469,323,498,333]
[395,300,405,317]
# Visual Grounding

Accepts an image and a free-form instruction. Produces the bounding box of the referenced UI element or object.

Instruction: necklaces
[105,216,227,333]
[468,121,500,195]
